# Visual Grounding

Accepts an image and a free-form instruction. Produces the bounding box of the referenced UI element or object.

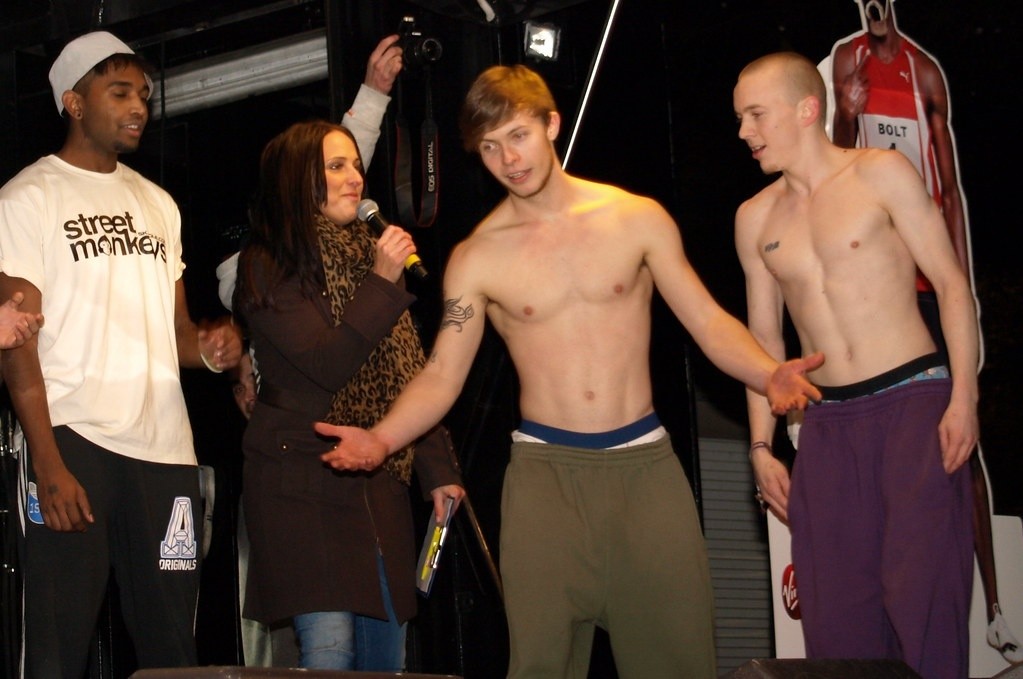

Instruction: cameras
[392,16,443,74]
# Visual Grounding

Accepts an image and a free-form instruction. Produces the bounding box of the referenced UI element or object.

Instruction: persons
[213,34,467,673]
[314,66,825,679]
[228,343,257,421]
[0,31,243,679]
[831,0,1023,667]
[734,52,981,679]
[0,292,44,349]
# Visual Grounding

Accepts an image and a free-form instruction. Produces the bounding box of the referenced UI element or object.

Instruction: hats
[48,30,154,118]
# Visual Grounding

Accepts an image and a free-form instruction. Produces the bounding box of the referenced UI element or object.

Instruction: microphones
[355,199,427,277]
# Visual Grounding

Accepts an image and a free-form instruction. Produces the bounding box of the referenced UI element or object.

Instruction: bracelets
[748,441,773,463]
[200,352,222,374]
